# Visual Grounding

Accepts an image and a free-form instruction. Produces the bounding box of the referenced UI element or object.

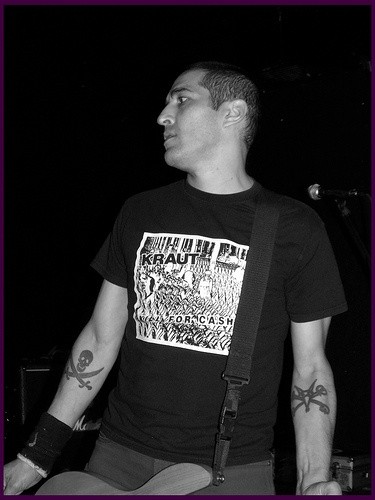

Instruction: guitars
[29,462,216,495]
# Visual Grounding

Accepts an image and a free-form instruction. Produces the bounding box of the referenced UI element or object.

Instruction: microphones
[308,184,367,200]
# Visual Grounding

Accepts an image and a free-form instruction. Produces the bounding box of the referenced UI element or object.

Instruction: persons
[0,57,343,497]
